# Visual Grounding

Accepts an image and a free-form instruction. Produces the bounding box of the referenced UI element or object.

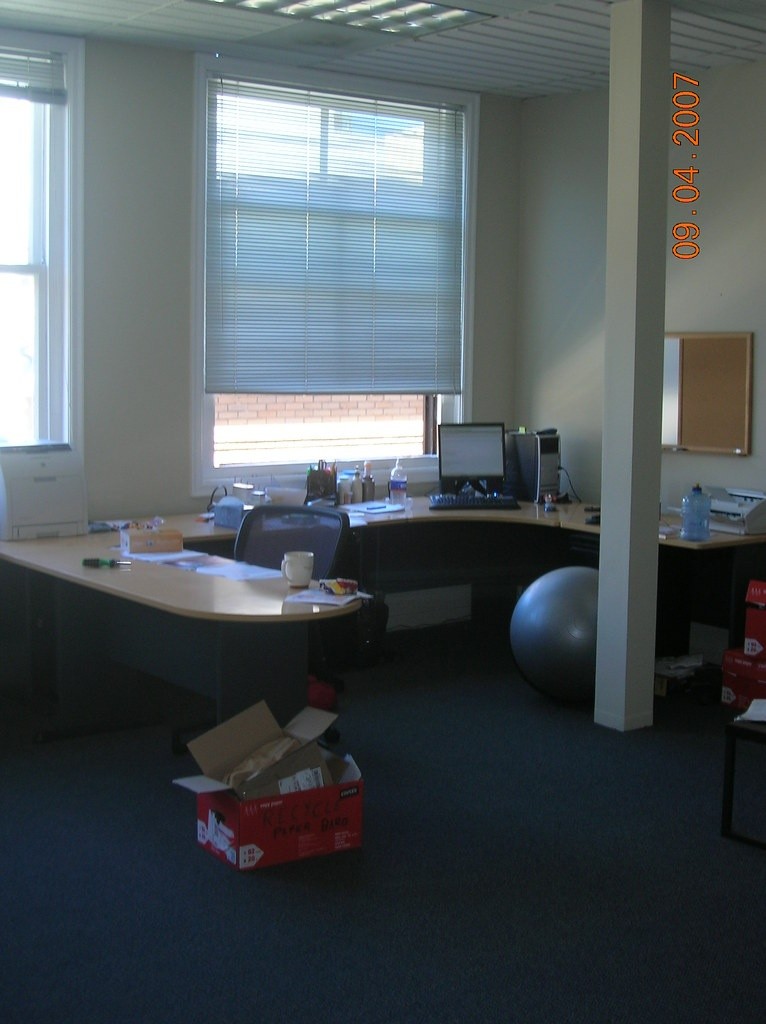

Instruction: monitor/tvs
[436,423,505,495]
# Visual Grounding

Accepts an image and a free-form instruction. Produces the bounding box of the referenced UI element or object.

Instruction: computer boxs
[506,429,560,503]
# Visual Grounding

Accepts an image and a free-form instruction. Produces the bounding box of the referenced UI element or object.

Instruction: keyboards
[428,493,521,510]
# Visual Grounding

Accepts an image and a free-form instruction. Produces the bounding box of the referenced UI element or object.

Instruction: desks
[0,494,766,720]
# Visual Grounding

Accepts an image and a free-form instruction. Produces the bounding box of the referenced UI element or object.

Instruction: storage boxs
[721,580,766,712]
[171,698,363,872]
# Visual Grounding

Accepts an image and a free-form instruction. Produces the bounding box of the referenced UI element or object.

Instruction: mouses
[544,502,556,512]
[544,493,555,502]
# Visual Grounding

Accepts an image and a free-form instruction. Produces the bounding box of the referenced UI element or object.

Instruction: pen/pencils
[308,459,336,472]
[367,506,386,510]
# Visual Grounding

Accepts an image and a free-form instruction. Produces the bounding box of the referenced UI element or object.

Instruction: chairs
[104,506,352,725]
[720,710,766,836]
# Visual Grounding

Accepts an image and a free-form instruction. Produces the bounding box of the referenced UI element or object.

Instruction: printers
[709,488,766,537]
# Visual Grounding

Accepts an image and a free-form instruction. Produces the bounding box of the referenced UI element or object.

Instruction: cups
[281,551,314,588]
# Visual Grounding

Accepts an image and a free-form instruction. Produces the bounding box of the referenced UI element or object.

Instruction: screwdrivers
[81,558,132,568]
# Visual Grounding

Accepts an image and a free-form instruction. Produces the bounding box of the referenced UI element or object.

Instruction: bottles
[353,464,361,480]
[338,478,351,505]
[390,458,408,506]
[352,470,362,502]
[362,461,375,502]
[681,484,711,541]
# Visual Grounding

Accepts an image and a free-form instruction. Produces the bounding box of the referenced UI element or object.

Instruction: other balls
[506,562,602,707]
